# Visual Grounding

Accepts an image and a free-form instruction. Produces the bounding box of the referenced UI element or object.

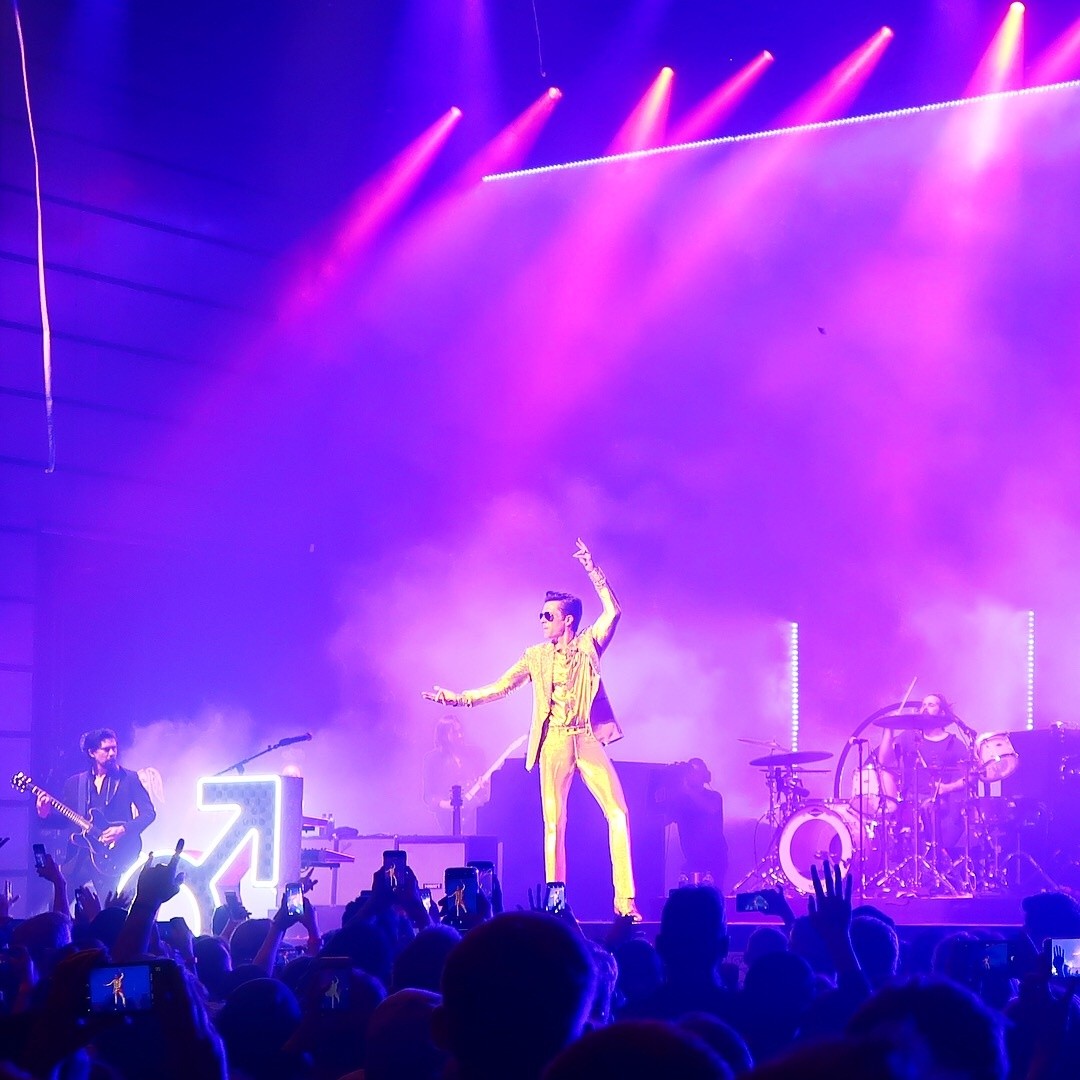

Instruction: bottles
[327,814,334,835]
[703,872,713,887]
[679,872,689,888]
[320,814,327,835]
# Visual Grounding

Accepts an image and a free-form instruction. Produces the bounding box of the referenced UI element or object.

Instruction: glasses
[538,612,554,621]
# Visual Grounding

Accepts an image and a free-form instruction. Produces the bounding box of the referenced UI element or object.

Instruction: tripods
[855,726,1059,896]
[729,747,778,895]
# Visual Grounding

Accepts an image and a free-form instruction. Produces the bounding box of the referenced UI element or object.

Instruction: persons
[420,538,640,923]
[0,839,1080,1079]
[647,758,728,890]
[879,693,975,854]
[136,768,164,808]
[35,729,156,908]
[424,714,491,834]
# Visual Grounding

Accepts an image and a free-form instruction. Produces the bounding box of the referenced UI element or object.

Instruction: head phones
[702,763,711,783]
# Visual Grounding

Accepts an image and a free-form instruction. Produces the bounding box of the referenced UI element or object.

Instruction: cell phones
[224,891,244,921]
[547,882,565,914]
[383,850,407,896]
[953,940,1023,976]
[467,861,495,920]
[736,891,776,912]
[420,888,431,916]
[1042,938,1080,985]
[444,866,479,931]
[317,957,354,1017]
[32,844,46,867]
[77,959,177,1014]
[276,948,297,966]
[285,882,305,921]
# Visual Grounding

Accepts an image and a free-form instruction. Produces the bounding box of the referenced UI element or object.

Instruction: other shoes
[613,905,643,922]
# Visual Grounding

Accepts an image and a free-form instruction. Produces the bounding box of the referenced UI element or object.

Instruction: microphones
[777,777,784,800]
[894,744,901,762]
[815,853,837,858]
[849,737,868,744]
[278,732,313,746]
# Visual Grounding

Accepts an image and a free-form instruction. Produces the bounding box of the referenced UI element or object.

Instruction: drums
[778,803,884,896]
[848,762,900,816]
[973,730,1022,782]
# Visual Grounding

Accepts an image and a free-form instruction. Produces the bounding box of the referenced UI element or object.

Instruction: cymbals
[748,751,834,766]
[872,714,954,729]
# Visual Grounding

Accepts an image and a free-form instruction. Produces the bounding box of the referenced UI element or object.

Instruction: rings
[584,550,587,554]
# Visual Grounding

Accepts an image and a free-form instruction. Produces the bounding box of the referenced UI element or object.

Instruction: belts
[548,725,587,735]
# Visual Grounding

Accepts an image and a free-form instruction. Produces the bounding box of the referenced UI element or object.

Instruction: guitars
[10,771,143,876]
